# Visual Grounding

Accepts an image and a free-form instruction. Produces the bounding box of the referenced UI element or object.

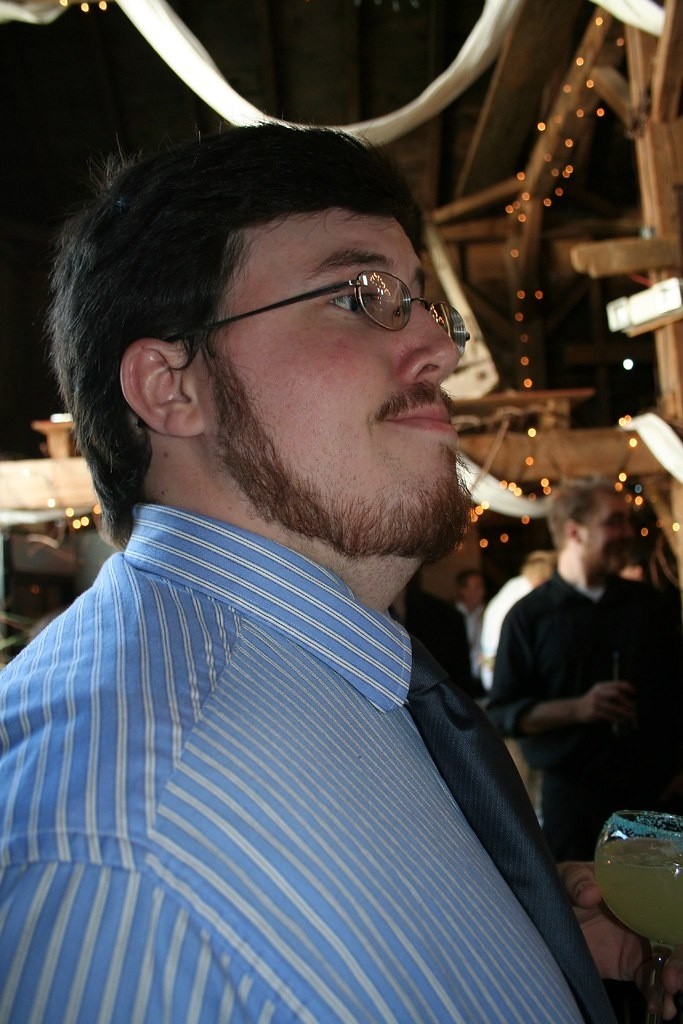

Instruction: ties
[404,634,619,1024]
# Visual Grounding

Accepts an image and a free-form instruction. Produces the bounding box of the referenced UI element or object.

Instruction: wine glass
[595,808,683,1023]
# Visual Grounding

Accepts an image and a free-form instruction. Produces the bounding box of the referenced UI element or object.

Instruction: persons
[0,122,683,1024]
[389,480,683,864]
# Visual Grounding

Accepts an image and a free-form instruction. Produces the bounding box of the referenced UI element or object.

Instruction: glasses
[137,269,470,428]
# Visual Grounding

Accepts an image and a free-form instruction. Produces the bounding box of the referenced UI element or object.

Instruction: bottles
[610,650,640,737]
